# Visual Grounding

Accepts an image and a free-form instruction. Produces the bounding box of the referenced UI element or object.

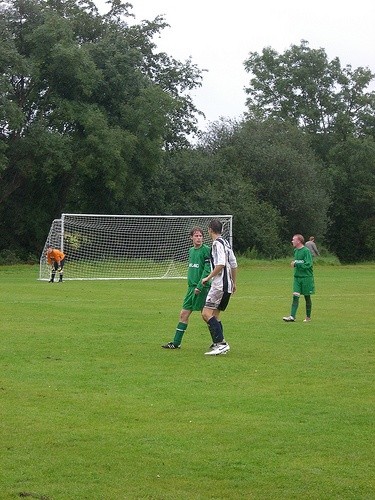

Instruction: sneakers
[210,344,217,349]
[162,342,181,349]
[204,341,230,355]
[303,317,311,322]
[283,316,294,322]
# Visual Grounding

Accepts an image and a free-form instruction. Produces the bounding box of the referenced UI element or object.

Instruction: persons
[201,220,238,356]
[282,234,315,322]
[305,236,321,257]
[161,226,217,350]
[47,246,65,283]
